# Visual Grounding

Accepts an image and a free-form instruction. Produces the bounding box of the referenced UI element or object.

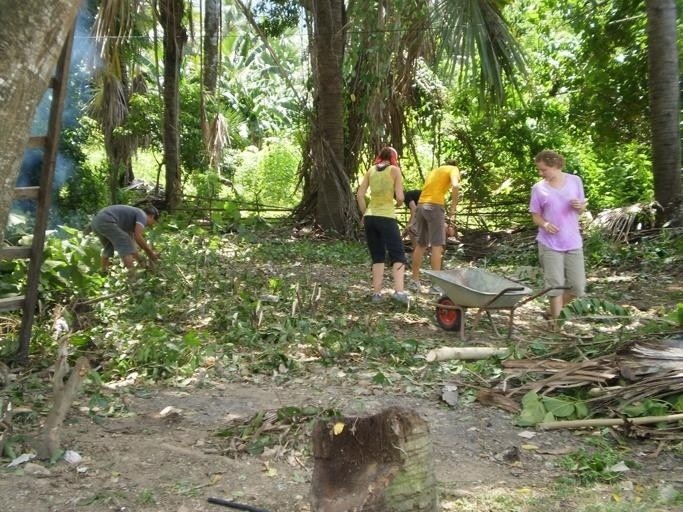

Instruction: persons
[528,151,587,326]
[410,158,459,296]
[356,147,414,304]
[394,187,421,247]
[91,205,159,278]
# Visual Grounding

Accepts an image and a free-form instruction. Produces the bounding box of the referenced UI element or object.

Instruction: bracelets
[543,221,549,230]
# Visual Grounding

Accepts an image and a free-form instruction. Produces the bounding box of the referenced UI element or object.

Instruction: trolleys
[424,266,574,341]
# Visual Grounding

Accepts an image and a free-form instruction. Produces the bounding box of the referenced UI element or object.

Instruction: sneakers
[372,278,444,305]
[541,308,561,333]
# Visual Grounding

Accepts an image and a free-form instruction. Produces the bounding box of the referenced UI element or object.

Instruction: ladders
[0,19,78,365]
